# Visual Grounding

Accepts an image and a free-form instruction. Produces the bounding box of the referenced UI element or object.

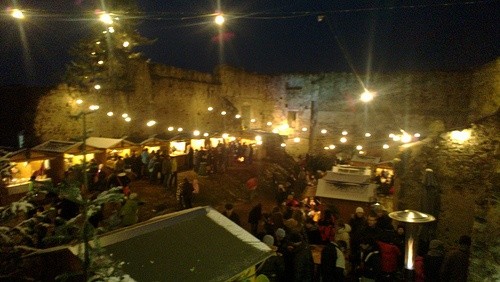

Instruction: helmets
[272,205,279,212]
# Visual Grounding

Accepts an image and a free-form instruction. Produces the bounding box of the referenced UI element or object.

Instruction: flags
[311,81,319,135]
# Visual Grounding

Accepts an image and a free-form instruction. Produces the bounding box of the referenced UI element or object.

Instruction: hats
[356,207,364,214]
[276,227,286,238]
[290,232,303,242]
[263,234,274,245]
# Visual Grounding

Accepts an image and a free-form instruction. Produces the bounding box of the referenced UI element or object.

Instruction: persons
[0,131,499,282]
